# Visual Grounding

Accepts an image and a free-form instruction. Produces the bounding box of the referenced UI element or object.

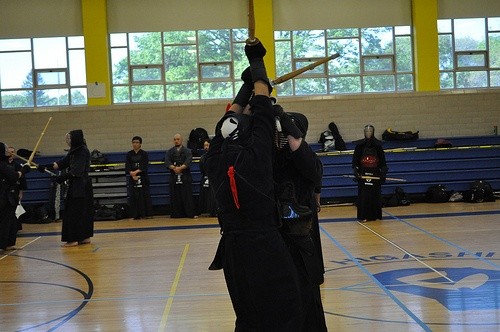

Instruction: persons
[36,129,95,247]
[0,142,31,255]
[200,39,327,332]
[165,133,199,218]
[352,124,388,222]
[125,136,149,221]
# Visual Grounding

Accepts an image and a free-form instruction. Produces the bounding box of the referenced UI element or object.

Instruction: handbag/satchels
[465,180,494,202]
[37,206,53,223]
[94,204,121,221]
[188,127,209,155]
[381,129,420,139]
[114,203,128,218]
[23,204,37,223]
[423,184,449,202]
[17,149,32,157]
[395,188,410,206]
[91,150,105,164]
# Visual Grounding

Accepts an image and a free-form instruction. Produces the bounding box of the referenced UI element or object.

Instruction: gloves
[244,41,273,94]
[273,104,303,139]
[353,166,359,181]
[232,67,254,109]
[379,167,390,183]
